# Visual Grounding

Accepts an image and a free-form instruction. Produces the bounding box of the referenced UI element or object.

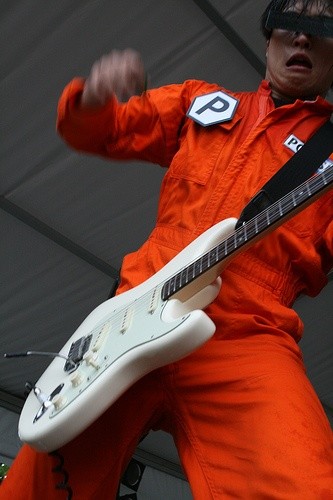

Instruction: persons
[0,0,332,500]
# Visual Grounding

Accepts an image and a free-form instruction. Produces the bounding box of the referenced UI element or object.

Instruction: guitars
[3,162,333,454]
